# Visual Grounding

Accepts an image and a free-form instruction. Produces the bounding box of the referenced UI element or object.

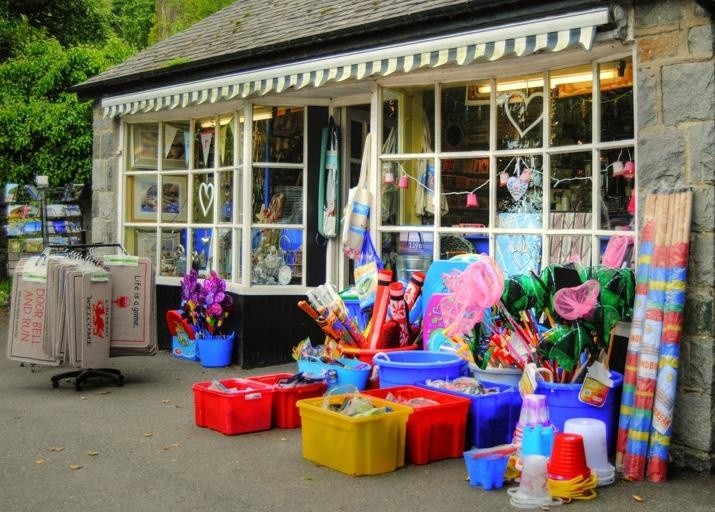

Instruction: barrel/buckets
[180,229,303,270]
[197,331,236,368]
[393,252,431,285]
[470,358,623,509]
[373,348,465,391]
[327,359,372,391]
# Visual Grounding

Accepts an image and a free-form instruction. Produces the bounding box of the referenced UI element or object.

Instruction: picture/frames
[128,121,190,262]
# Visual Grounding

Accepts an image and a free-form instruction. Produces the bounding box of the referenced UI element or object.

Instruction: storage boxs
[292,391,415,478]
[414,373,522,456]
[357,383,472,467]
[191,371,276,436]
[248,372,329,430]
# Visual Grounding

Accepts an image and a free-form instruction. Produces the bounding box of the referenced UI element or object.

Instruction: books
[3,182,83,277]
